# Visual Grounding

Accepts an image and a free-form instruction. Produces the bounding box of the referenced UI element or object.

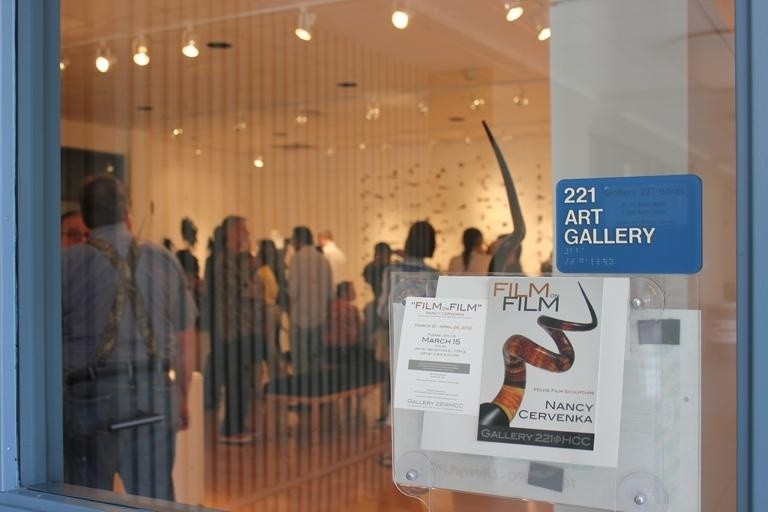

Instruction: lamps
[59,0,552,73]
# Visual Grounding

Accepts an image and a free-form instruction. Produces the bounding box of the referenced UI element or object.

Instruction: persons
[165,214,366,443]
[61,172,201,503]
[361,220,529,327]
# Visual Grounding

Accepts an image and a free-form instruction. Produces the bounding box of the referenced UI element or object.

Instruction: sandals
[377,454,392,467]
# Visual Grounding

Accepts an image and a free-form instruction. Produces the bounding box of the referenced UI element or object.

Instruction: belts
[67,360,170,383]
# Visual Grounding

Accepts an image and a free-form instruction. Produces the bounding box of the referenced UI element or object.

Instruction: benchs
[263,364,389,445]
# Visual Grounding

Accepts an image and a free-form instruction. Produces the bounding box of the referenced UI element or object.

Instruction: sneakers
[219,432,255,444]
